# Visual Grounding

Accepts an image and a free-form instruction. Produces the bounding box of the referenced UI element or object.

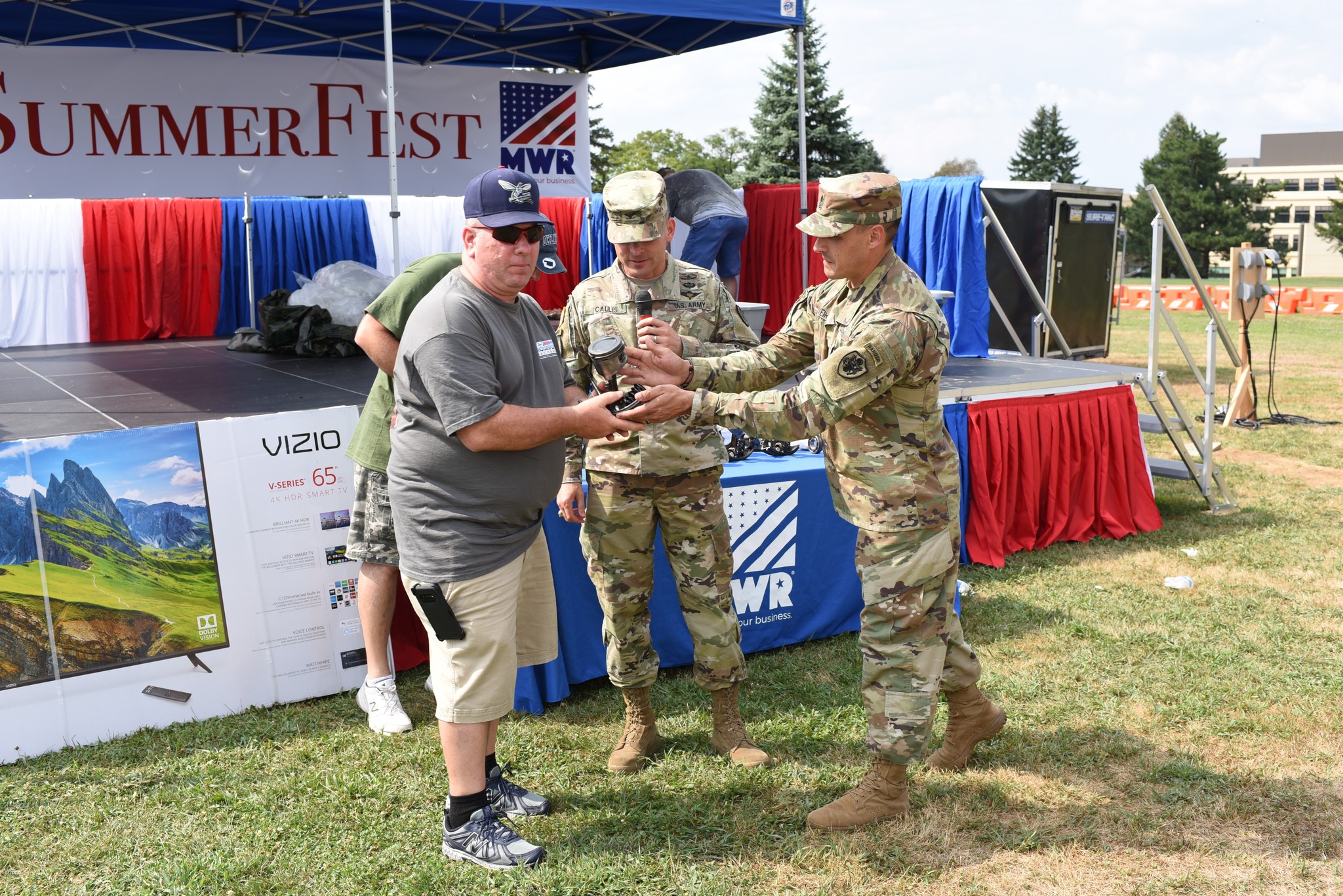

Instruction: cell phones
[415,582,464,640]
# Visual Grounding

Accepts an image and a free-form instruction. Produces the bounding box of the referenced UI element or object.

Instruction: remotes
[141,685,192,702]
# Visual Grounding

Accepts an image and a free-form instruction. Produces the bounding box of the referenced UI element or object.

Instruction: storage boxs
[983,180,1126,361]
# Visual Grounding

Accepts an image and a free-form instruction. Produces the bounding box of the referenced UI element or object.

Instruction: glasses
[467,224,545,245]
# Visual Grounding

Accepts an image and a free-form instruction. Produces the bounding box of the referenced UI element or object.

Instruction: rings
[654,335,656,343]
[558,510,564,517]
[645,420,650,424]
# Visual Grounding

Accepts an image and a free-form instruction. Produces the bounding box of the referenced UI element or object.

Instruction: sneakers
[440,804,544,872]
[355,673,413,737]
[424,674,434,693]
[440,761,551,818]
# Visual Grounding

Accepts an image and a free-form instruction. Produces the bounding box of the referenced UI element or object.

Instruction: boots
[806,757,912,835]
[606,685,663,774]
[709,683,770,770]
[925,682,1008,773]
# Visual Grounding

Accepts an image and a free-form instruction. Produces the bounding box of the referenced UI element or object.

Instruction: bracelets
[679,358,693,386]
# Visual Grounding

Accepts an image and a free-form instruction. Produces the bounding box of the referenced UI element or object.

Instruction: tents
[0,0,809,292]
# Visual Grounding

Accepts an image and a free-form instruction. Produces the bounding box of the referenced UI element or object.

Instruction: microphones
[635,290,655,344]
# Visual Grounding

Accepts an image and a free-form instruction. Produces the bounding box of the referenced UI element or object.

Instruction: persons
[556,170,770,775]
[386,165,646,872]
[656,167,749,301]
[613,169,1007,836]
[344,211,567,737]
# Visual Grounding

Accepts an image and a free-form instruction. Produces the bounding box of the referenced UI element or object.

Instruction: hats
[601,169,669,245]
[794,172,903,239]
[463,166,555,229]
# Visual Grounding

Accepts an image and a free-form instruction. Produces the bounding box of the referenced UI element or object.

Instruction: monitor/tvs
[0,421,230,691]
